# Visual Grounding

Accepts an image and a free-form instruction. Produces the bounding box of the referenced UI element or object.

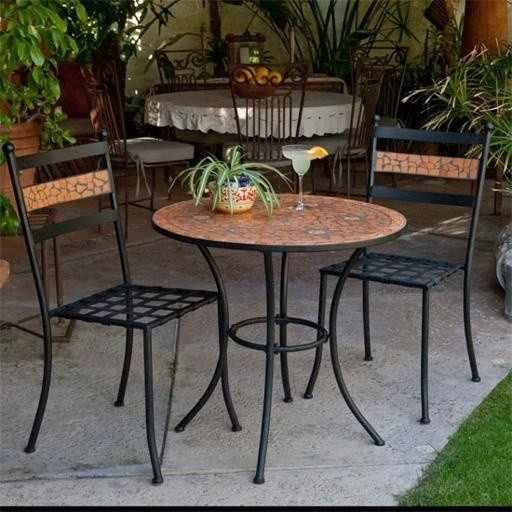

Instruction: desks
[152,192,409,485]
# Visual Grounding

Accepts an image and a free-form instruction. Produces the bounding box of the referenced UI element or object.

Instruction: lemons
[309,146,328,160]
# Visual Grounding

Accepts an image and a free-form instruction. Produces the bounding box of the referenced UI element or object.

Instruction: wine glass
[283,144,319,214]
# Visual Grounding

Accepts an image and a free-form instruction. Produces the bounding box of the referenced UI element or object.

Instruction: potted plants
[0,1,79,229]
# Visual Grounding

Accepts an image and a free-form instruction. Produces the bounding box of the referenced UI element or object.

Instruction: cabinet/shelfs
[2,205,66,327]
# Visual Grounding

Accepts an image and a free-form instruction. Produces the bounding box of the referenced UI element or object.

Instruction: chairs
[3,140,244,486]
[87,30,410,241]
[303,114,492,424]
